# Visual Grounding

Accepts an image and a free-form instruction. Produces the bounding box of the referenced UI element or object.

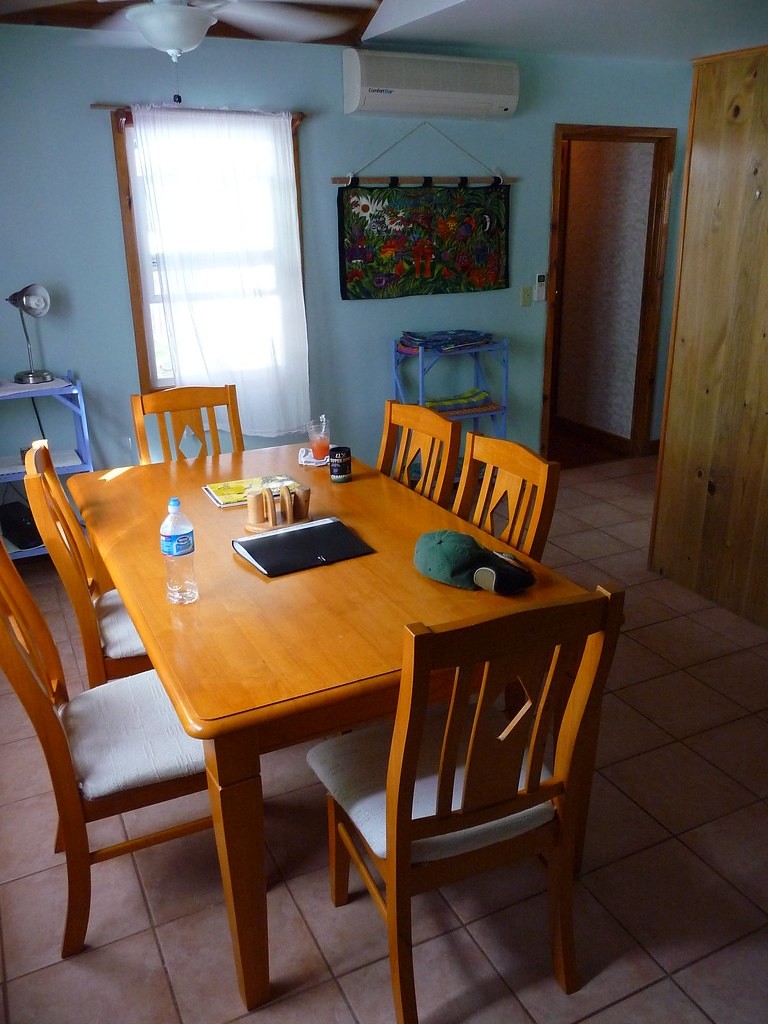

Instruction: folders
[231,515,375,578]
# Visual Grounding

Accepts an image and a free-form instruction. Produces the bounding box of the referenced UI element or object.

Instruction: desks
[65,441,594,1008]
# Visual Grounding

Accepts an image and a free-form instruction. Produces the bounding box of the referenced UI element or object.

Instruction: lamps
[5,283,54,383]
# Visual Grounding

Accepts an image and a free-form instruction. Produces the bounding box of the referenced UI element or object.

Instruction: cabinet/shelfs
[645,44,768,630]
[392,339,510,483]
[0,370,93,559]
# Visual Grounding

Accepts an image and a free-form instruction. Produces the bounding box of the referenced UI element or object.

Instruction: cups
[307,418,331,460]
[329,446,352,483]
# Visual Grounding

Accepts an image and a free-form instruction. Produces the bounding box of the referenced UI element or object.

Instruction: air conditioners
[343,48,521,119]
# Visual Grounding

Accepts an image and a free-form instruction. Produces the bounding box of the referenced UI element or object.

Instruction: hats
[413,530,536,595]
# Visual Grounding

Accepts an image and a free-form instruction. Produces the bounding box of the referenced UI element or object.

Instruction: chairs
[340,432,560,736]
[23,437,155,690]
[0,538,266,957]
[373,399,463,509]
[304,581,624,1024]
[132,383,245,465]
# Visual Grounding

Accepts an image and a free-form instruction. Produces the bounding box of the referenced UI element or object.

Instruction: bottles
[160,496,200,605]
